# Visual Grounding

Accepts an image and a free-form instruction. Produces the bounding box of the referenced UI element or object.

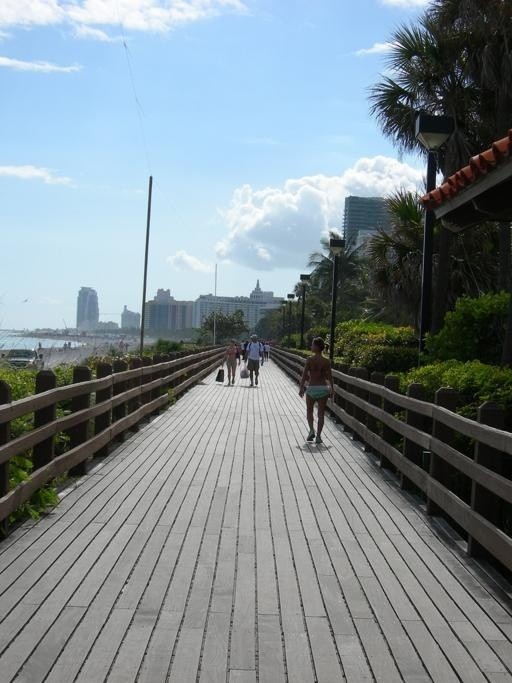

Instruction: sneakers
[306,430,323,443]
[227,378,258,386]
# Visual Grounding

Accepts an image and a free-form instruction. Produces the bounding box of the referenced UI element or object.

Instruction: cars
[1,348,44,371]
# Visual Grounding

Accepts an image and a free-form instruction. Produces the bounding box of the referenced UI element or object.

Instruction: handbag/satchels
[215,364,224,382]
[239,369,250,378]
[235,347,240,358]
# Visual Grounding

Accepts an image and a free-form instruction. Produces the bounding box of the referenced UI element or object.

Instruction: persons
[299,336,336,443]
[221,338,240,385]
[244,333,266,387]
[235,338,271,362]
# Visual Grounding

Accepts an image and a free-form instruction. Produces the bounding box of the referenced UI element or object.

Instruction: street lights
[330,239,345,357]
[300,275,310,350]
[280,294,295,348]
[414,112,454,366]
